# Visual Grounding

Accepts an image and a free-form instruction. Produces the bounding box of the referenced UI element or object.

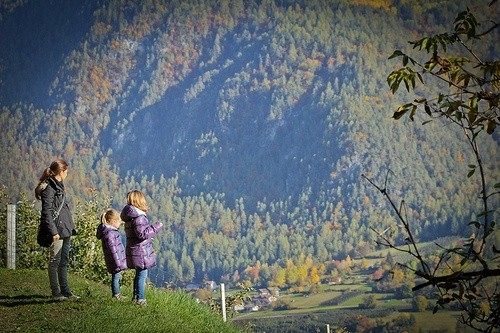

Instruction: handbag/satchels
[36,224,53,247]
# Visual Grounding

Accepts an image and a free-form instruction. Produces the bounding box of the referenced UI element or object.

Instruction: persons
[120,190,163,306]
[96,209,129,301]
[34,158,82,300]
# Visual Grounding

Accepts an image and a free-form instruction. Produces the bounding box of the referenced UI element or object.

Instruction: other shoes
[66,292,82,301]
[112,293,127,301]
[132,298,148,307]
[51,296,68,301]
